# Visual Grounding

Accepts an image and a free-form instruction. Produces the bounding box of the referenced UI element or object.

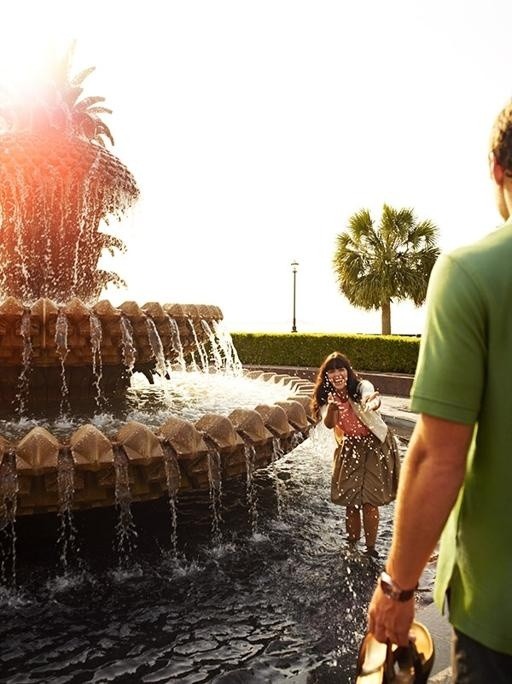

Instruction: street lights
[290,258,300,333]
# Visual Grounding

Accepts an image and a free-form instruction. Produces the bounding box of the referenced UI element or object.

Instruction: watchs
[379,571,421,604]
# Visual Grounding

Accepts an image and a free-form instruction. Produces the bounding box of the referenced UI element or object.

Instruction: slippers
[353,619,435,684]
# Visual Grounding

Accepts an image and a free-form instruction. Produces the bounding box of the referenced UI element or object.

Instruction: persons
[311,352,401,552]
[359,96,511,682]
